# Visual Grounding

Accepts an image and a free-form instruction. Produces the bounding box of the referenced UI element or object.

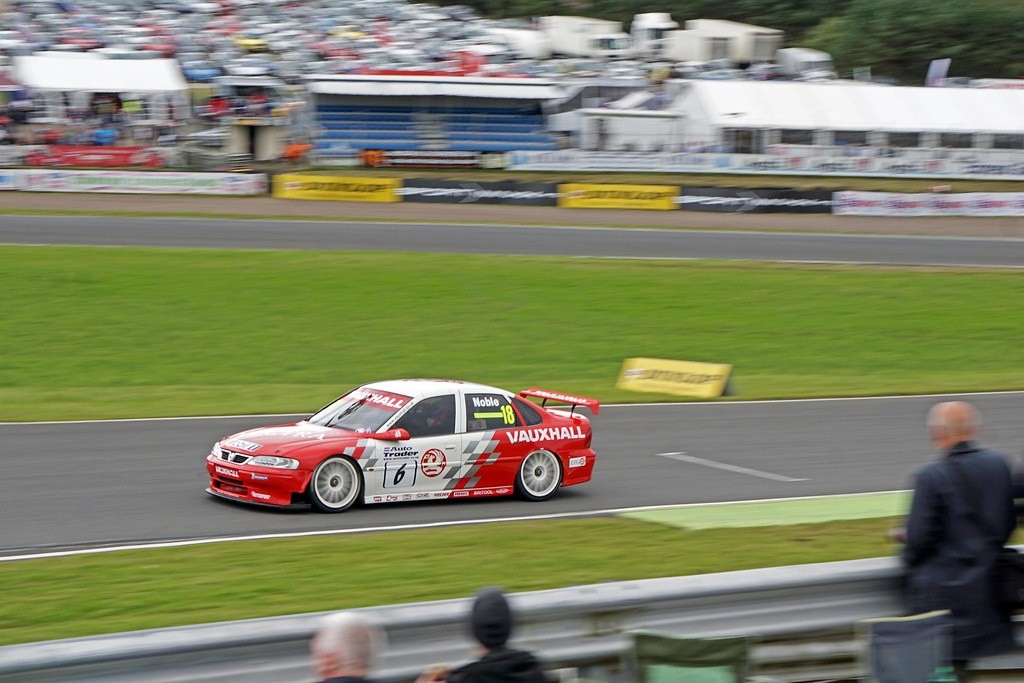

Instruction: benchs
[317,102,561,153]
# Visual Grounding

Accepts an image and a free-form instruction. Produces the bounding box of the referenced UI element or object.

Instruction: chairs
[626,627,753,682]
[413,402,430,420]
[861,606,958,683]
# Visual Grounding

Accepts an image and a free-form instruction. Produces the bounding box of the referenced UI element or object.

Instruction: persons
[887,401,1019,683]
[403,401,454,435]
[308,611,375,683]
[413,588,560,683]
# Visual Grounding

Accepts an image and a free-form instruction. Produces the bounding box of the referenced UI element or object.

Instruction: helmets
[427,400,457,429]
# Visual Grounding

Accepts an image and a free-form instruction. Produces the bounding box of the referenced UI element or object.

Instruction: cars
[202,376,601,516]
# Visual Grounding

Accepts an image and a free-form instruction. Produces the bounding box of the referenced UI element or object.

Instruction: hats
[472,588,513,639]
